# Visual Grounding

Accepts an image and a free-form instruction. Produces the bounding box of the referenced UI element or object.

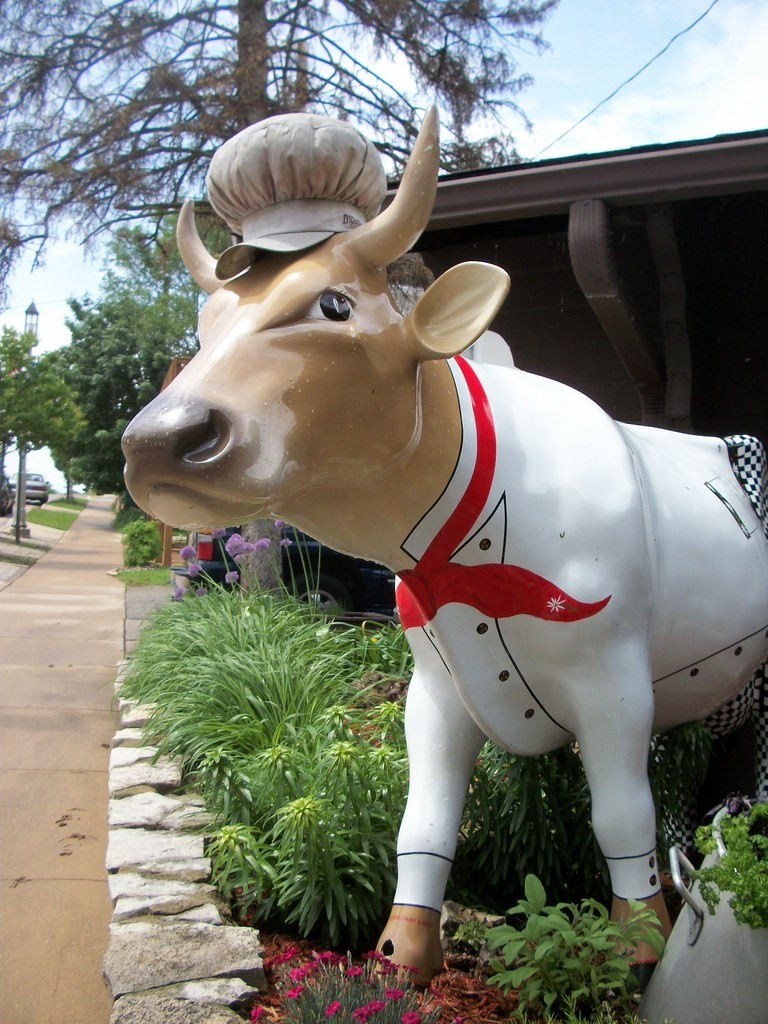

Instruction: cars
[170,514,399,627]
[10,472,49,504]
[0,474,17,514]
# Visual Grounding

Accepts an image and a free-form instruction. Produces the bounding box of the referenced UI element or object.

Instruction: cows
[119,105,768,994]
